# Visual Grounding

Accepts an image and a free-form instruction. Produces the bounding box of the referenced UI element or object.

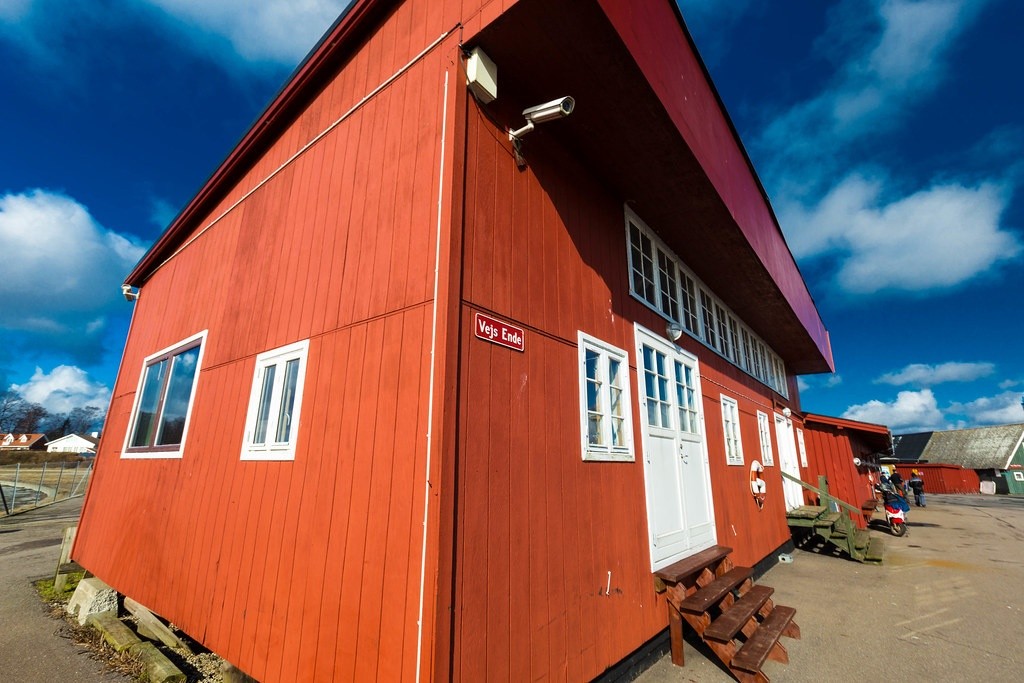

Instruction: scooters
[875,484,911,536]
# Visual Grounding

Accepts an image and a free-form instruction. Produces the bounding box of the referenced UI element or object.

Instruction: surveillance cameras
[122,284,134,302]
[522,96,575,124]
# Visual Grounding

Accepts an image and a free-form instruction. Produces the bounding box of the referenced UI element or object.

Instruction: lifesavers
[749,459,768,504]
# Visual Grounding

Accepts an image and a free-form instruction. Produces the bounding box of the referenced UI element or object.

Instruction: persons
[889,468,909,502]
[879,471,890,484]
[908,472,927,507]
